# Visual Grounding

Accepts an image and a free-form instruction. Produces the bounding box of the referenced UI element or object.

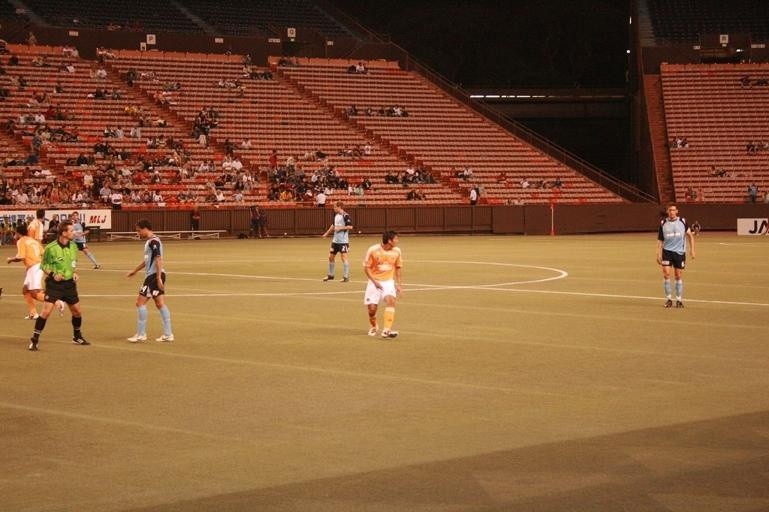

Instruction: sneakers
[25,313,39,320]
[156,333,173,342]
[128,333,147,342]
[71,336,90,345]
[343,277,350,282]
[29,342,38,350]
[323,274,334,281]
[55,300,65,318]
[676,301,684,308]
[368,324,379,336]
[381,329,398,338]
[664,300,674,307]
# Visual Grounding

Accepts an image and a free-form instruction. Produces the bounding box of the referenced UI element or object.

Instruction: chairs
[633,0,768,208]
[1,1,624,211]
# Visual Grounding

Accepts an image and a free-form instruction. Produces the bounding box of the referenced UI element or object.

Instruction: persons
[464,167,478,205]
[126,220,175,343]
[384,164,434,202]
[348,104,409,117]
[654,201,696,309]
[356,62,366,73]
[278,54,298,67]
[261,69,272,79]
[71,212,101,270]
[2,31,260,204]
[669,72,769,203]
[362,230,403,339]
[691,220,701,235]
[191,205,199,230]
[320,202,355,282]
[2,214,59,245]
[28,220,91,351]
[265,141,373,203]
[6,224,64,320]
[520,175,561,189]
[248,205,269,240]
